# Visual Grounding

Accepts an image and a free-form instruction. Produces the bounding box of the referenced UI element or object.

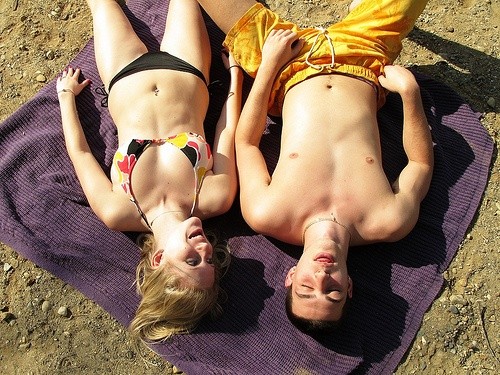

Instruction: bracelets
[58,88,76,96]
[227,65,242,70]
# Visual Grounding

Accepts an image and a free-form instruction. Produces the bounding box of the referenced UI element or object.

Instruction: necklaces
[150,210,188,226]
[302,212,352,244]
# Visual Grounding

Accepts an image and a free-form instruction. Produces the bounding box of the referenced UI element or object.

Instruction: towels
[0,0,495,372]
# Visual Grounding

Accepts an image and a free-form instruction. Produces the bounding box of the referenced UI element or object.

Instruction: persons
[55,0,245,346]
[198,0,436,337]
